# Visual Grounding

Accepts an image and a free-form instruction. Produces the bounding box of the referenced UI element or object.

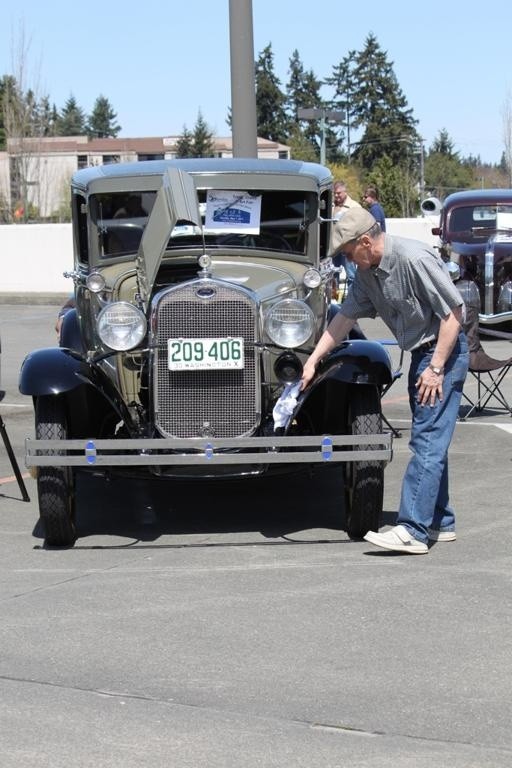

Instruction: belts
[410,335,436,354]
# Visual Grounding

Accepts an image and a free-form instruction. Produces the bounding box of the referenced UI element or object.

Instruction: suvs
[13,156,400,549]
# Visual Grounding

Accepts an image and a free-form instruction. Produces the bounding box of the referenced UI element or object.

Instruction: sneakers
[428,528,457,542]
[363,525,429,554]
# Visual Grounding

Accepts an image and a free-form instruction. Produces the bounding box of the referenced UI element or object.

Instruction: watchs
[428,362,445,375]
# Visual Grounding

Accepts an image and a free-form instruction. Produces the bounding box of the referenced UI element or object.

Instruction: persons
[363,188,386,233]
[298,206,470,554]
[332,179,356,305]
[55,296,76,348]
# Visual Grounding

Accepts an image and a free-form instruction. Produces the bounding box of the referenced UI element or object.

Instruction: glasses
[341,234,368,257]
[363,195,368,199]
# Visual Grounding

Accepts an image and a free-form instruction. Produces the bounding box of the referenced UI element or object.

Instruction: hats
[326,206,377,258]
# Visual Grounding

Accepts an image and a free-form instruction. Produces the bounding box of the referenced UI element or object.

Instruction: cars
[433,189,511,334]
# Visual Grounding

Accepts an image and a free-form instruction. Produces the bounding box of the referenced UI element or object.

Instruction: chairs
[458,307,512,422]
[0,336,30,502]
